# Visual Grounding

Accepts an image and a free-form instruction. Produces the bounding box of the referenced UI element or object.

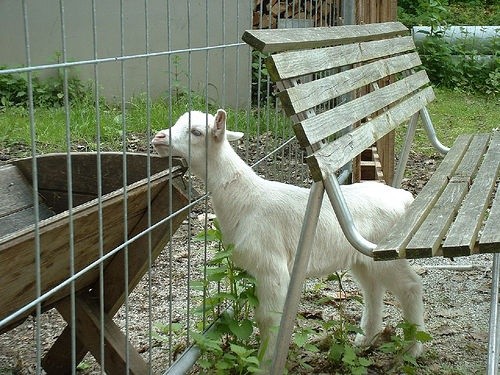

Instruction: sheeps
[151,109,424,375]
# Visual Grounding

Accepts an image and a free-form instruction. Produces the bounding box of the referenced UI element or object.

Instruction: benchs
[242,22,500,375]
